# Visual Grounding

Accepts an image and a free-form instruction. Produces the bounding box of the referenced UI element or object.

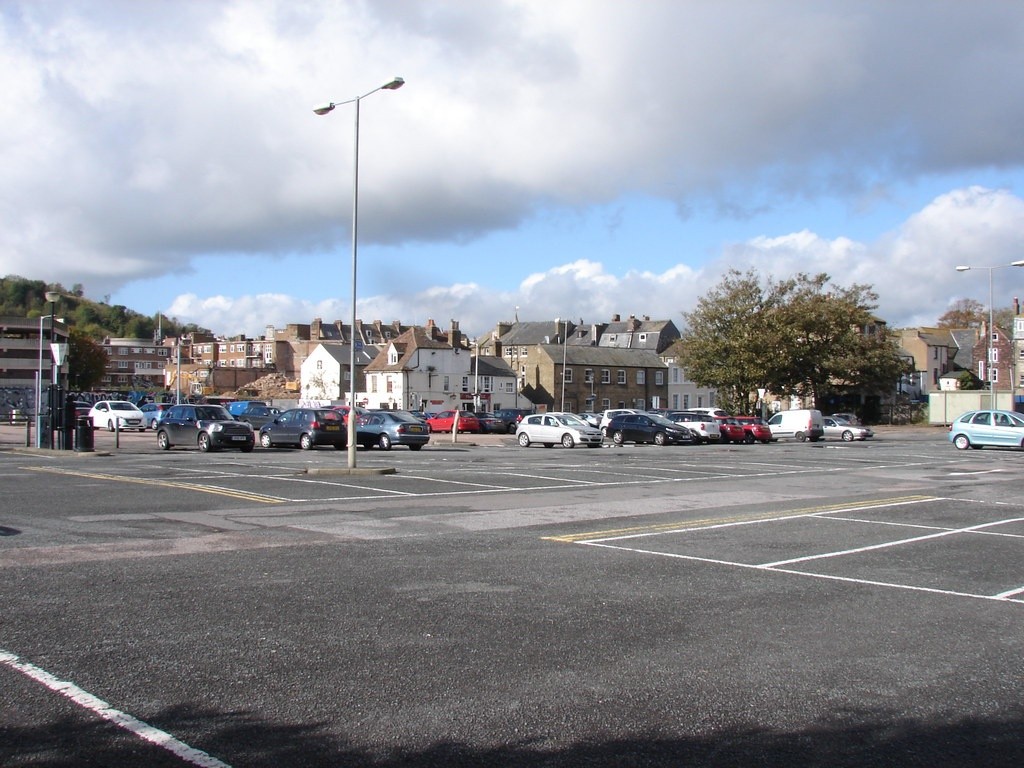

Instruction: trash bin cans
[74,414,95,452]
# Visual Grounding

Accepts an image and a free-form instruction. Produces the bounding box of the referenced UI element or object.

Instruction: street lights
[469,341,479,413]
[555,318,568,412]
[45,291,61,383]
[38,315,56,448]
[311,75,406,467]
[955,259,1024,411]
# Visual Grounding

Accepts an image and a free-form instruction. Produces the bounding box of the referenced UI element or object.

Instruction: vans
[767,409,823,442]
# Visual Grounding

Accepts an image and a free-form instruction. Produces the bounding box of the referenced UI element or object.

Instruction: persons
[516,415,523,423]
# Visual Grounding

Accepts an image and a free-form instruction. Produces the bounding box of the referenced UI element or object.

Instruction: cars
[426,409,480,434]
[140,403,174,430]
[833,413,862,426]
[649,407,726,445]
[606,414,692,445]
[546,412,603,428]
[87,401,147,433]
[734,417,771,444]
[818,417,874,442]
[238,406,285,430]
[599,409,649,437]
[157,404,256,453]
[73,401,100,430]
[515,413,603,448]
[714,416,744,444]
[948,410,1024,450]
[356,409,430,451]
[320,405,370,423]
[494,409,537,434]
[473,412,504,434]
[259,408,347,450]
